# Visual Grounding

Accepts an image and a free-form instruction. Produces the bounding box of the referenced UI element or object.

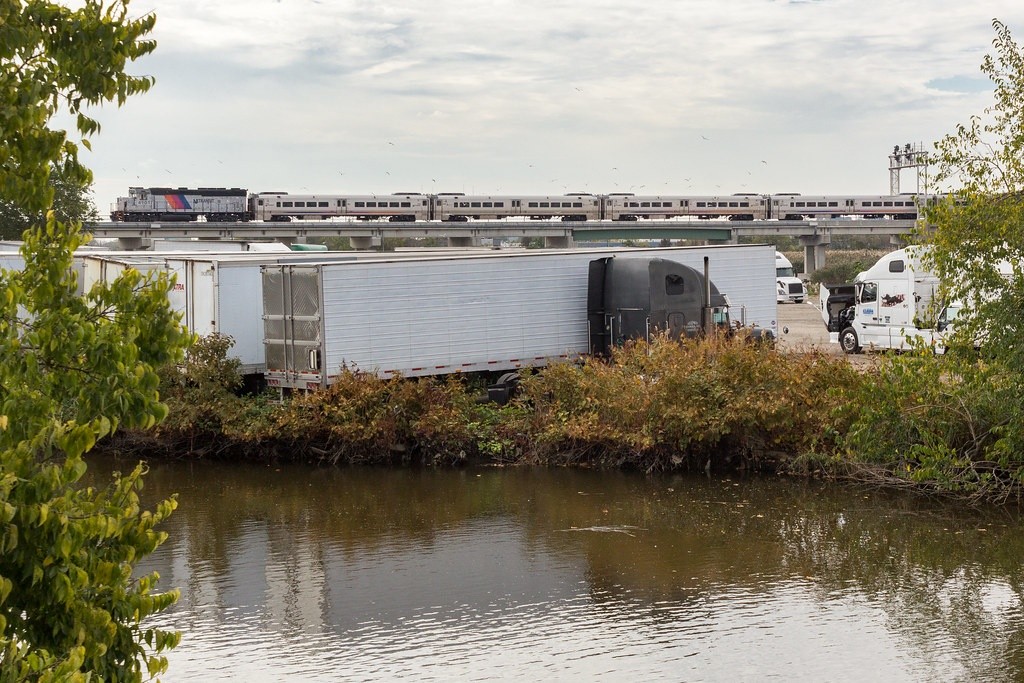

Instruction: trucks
[776,251,808,305]
[0,227,778,402]
[817,242,1024,357]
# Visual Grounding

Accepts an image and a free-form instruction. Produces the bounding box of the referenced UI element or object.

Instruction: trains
[109,184,979,227]
